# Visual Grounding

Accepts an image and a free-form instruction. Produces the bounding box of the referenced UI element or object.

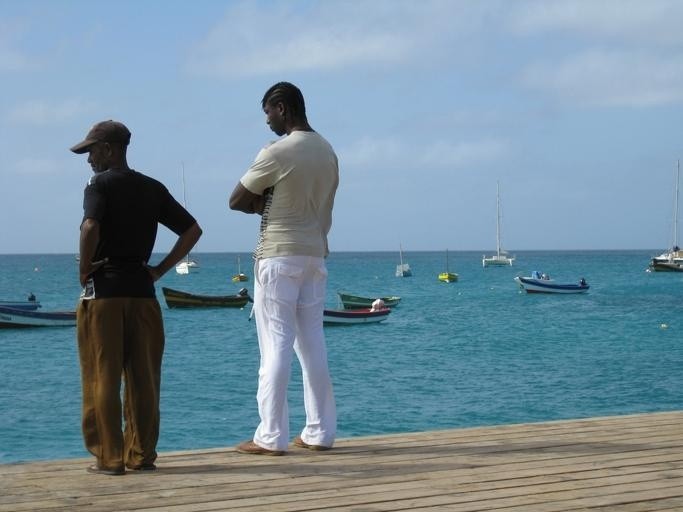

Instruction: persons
[227,81,340,458]
[68,117,204,477]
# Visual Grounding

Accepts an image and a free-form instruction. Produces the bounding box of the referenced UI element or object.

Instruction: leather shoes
[235,439,283,455]
[293,435,327,450]
[87,465,125,475]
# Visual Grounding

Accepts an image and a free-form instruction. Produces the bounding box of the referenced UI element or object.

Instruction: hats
[71,120,131,154]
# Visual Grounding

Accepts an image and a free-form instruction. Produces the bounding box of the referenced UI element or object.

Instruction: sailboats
[437,247,457,284]
[481,176,514,269]
[393,239,411,277]
[647,155,682,274]
[229,253,248,283]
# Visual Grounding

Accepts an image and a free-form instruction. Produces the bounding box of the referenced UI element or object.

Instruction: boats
[512,269,590,294]
[159,285,253,310]
[0,303,78,329]
[322,302,392,326]
[336,291,403,311]
[0,290,44,310]
[175,251,202,276]
